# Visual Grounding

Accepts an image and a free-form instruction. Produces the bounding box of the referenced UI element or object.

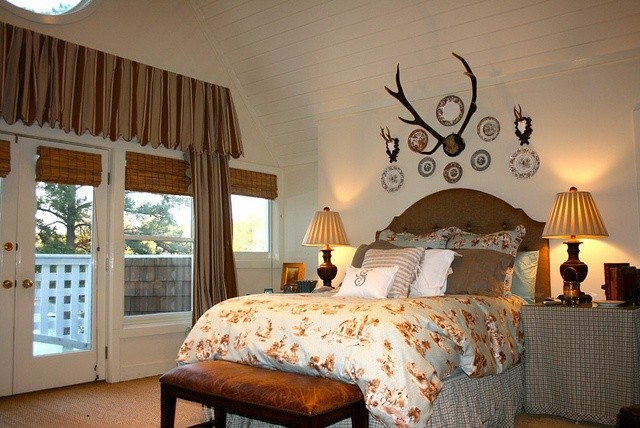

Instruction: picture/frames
[281,263,304,290]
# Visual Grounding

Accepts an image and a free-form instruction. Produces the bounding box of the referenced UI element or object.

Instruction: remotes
[543,300,562,305]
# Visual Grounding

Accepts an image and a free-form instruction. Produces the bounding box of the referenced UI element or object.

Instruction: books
[604,263,639,301]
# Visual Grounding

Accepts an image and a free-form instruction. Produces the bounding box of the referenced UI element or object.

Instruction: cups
[561,280,581,306]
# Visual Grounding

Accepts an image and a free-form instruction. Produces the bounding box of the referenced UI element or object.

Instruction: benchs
[159,359,370,428]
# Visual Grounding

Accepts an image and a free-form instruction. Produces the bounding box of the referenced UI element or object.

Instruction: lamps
[541,186,609,303]
[301,206,350,289]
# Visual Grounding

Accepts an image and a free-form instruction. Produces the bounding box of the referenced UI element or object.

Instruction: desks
[176,189,551,428]
[520,301,640,426]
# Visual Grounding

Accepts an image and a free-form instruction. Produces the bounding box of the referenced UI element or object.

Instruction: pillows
[446,225,526,297]
[378,226,448,248]
[332,263,399,299]
[408,248,463,298]
[351,240,402,268]
[362,247,425,298]
[510,250,540,305]
[445,248,516,297]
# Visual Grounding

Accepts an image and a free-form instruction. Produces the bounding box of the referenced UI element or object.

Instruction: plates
[595,300,626,307]
